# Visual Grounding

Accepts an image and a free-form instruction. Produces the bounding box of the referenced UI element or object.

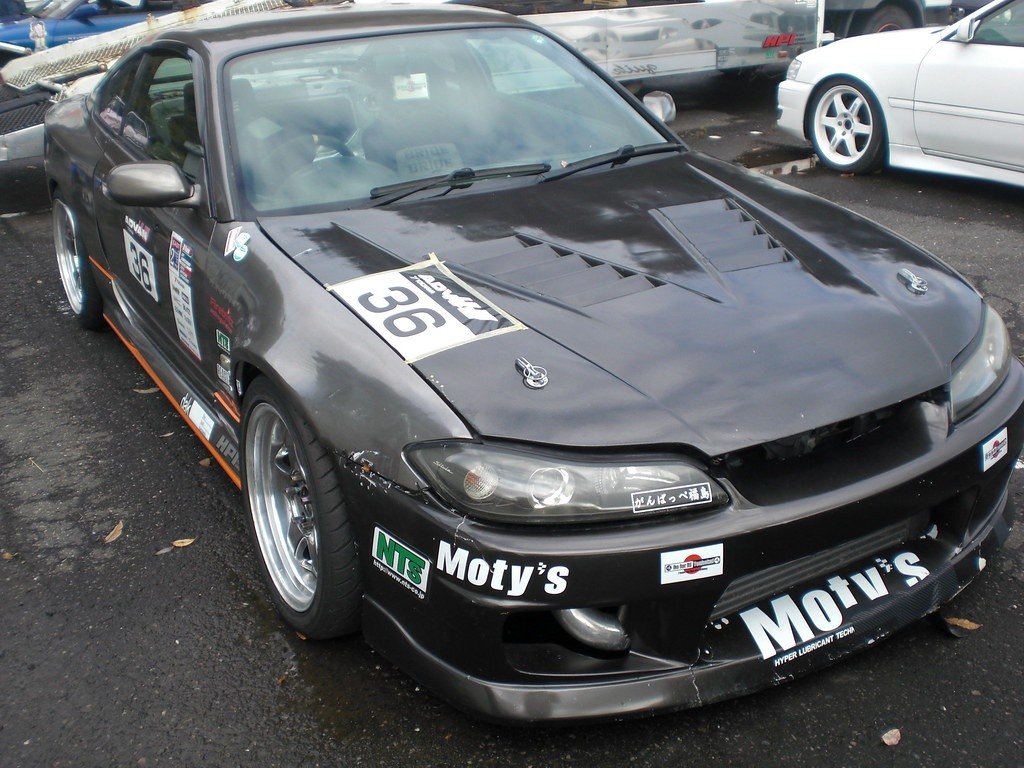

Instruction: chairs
[364,59,493,171]
[170,78,316,179]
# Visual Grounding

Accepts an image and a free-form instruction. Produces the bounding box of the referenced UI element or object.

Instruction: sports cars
[42,3,1024,722]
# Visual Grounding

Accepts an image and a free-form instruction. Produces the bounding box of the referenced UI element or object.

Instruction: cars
[776,0,1024,190]
[0,1,212,56]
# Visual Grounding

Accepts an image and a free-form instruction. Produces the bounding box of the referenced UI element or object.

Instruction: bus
[466,0,953,98]
